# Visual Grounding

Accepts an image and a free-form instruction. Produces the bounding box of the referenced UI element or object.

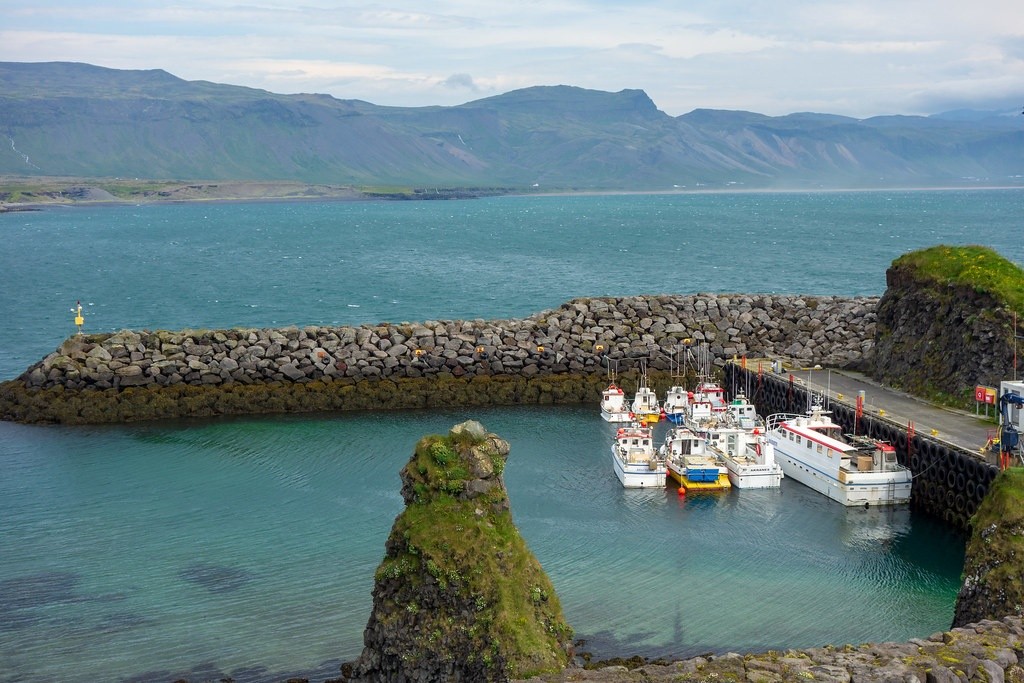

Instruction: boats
[700,392,785,489]
[631,358,661,422]
[598,353,633,422]
[682,340,766,435]
[659,425,733,490]
[766,369,915,508]
[663,343,690,424]
[610,423,670,490]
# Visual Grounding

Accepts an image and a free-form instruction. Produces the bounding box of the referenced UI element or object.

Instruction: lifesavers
[758,375,1001,533]
[755,443,762,457]
